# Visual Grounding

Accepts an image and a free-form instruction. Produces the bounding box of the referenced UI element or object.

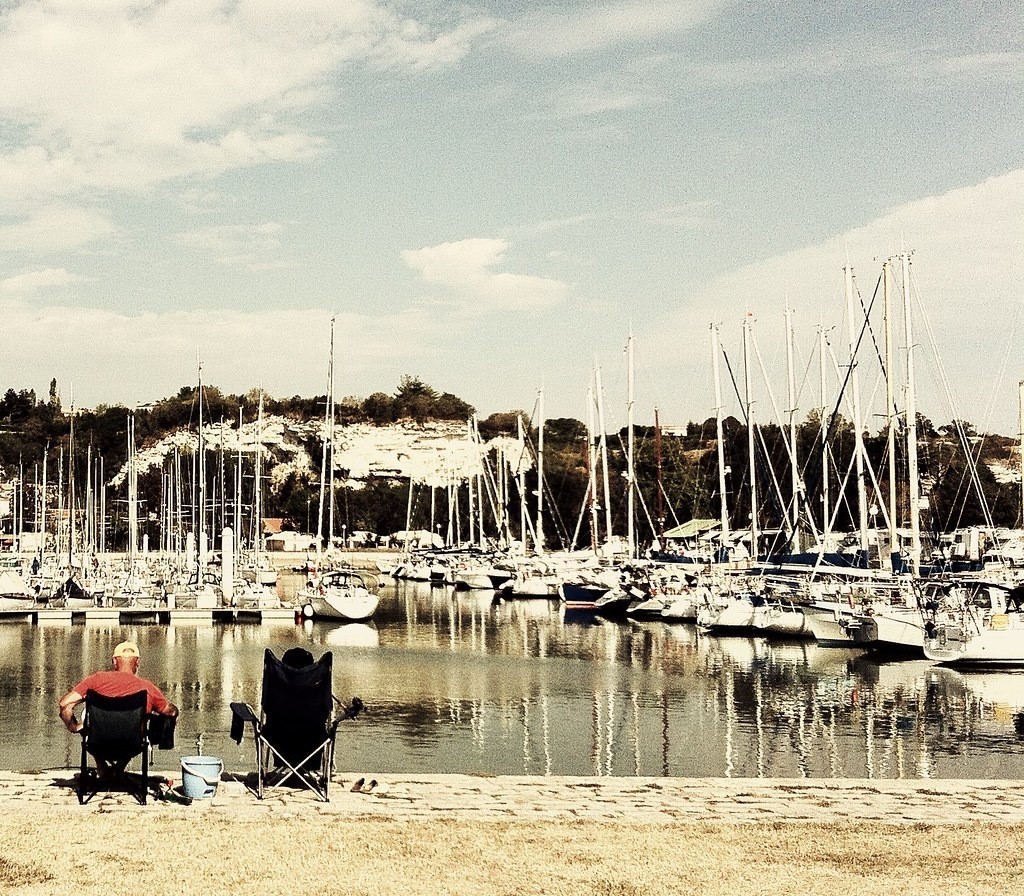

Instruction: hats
[114,641,139,657]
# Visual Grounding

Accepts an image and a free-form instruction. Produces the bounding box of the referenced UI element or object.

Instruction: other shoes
[350,778,365,792]
[361,780,378,793]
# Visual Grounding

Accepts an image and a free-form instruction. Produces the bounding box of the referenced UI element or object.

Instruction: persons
[58,643,180,780]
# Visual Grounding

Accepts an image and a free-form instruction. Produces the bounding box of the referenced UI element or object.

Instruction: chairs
[77,689,157,806]
[229,648,339,803]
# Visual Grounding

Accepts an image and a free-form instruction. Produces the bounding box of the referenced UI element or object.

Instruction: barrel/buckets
[179,756,224,798]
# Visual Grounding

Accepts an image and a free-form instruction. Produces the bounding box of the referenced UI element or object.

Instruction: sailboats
[2,246,1024,670]
